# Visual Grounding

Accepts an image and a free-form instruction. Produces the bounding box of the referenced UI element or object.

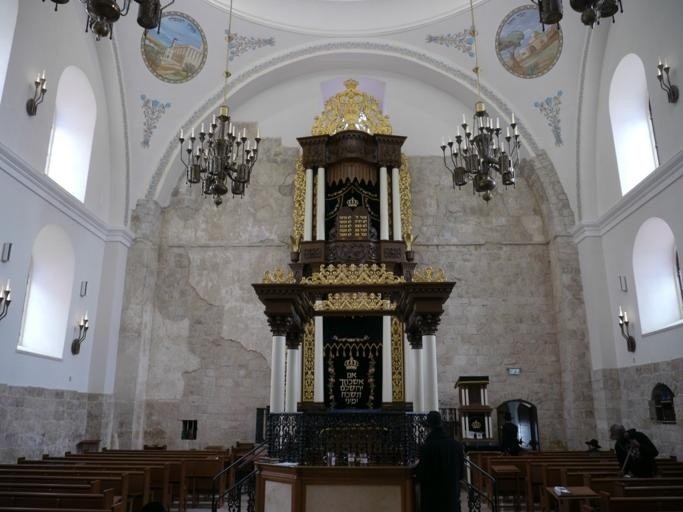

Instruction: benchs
[0,443,255,512]
[465,451,682,512]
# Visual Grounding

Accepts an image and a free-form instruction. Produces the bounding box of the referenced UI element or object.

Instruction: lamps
[615,304,637,355]
[0,279,14,320]
[177,2,260,208]
[41,0,174,41]
[26,71,48,117]
[655,53,679,107]
[70,312,89,354]
[439,2,521,202]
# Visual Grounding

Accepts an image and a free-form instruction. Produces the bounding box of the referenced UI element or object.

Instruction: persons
[610,423,658,477]
[502,412,520,451]
[585,440,602,452]
[415,410,465,512]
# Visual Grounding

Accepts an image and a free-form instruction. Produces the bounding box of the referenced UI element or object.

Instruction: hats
[610,424,625,440]
[585,439,601,448]
[425,410,441,424]
[502,412,514,419]
